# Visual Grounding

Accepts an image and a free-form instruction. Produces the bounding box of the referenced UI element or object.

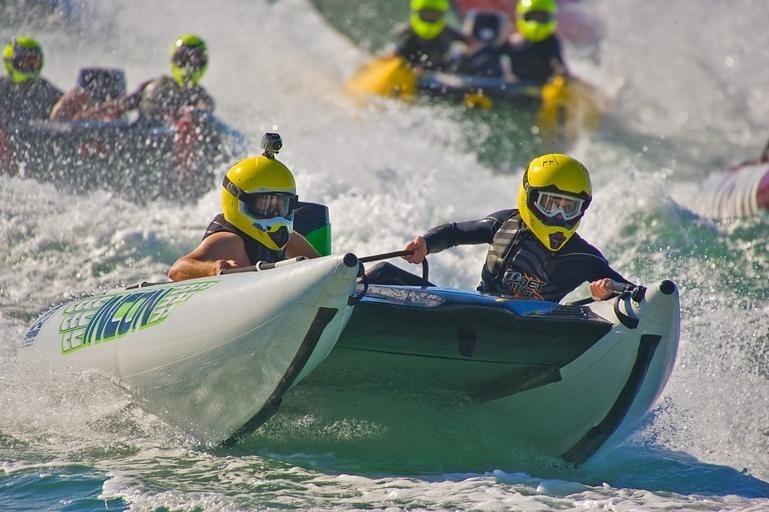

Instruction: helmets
[514,0,559,43]
[219,155,298,254]
[167,35,210,90]
[517,153,594,253]
[4,35,46,86]
[407,0,451,41]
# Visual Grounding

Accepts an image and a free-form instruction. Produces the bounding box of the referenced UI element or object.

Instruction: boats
[2,114,243,205]
[19,252,680,473]
[697,169,765,216]
[338,53,608,177]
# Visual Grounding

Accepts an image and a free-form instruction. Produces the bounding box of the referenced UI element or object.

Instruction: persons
[168,156,322,282]
[401,154,635,303]
[0,33,215,131]
[393,0,564,84]
